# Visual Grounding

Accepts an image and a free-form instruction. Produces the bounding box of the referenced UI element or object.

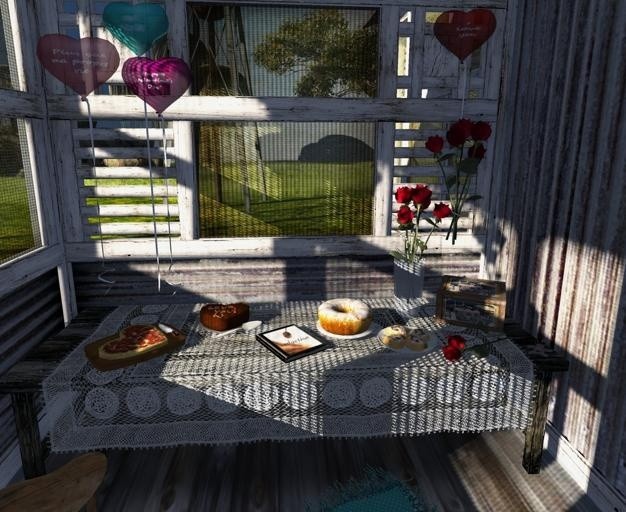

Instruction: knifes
[158,323,185,343]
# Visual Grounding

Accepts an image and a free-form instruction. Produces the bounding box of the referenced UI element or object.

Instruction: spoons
[213,321,261,337]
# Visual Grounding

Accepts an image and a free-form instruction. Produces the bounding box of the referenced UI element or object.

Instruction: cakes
[383,325,429,351]
[100,323,169,363]
[199,299,250,332]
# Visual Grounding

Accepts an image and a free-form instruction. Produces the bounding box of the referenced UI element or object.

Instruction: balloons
[102,2,169,56]
[34,33,122,103]
[121,56,193,119]
[433,7,497,66]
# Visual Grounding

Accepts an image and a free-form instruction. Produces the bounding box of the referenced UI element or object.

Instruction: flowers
[396,114,494,303]
[440,331,515,363]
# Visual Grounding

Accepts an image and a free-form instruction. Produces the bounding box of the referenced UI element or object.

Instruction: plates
[84,334,174,371]
[316,319,376,339]
[377,325,438,355]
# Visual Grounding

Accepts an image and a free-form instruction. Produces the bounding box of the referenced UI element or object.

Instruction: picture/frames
[255,323,333,364]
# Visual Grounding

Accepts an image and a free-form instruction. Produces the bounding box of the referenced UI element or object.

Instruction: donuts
[316,296,373,334]
